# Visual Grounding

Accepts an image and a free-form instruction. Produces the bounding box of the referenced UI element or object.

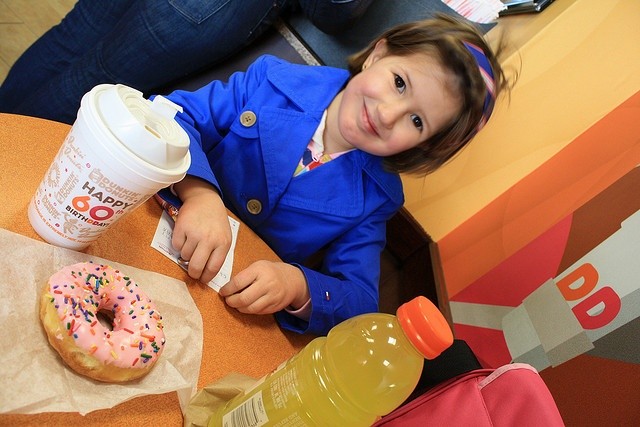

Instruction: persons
[147,10,524,337]
[0,0,298,126]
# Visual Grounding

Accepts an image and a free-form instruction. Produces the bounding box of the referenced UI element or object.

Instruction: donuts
[38,262,165,384]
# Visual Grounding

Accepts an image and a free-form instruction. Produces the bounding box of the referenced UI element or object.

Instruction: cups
[27,83,191,252]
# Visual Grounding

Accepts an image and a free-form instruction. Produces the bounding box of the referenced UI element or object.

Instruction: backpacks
[370,363,565,427]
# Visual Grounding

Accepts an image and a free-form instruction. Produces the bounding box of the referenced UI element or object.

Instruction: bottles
[207,295,455,427]
